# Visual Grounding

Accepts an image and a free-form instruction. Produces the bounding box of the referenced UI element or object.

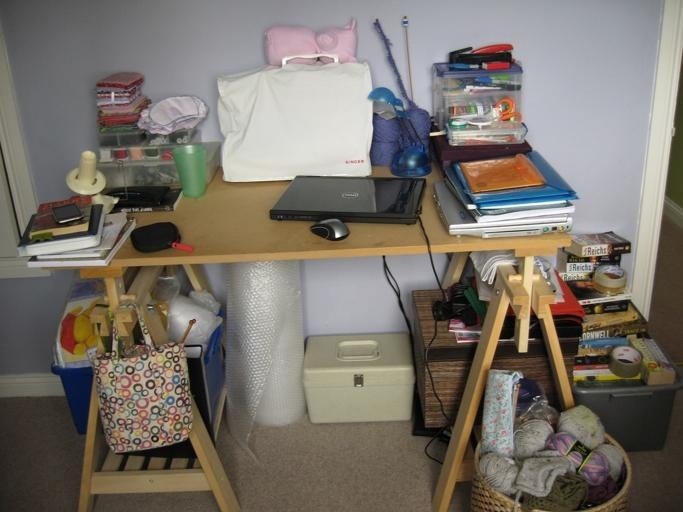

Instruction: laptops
[270,176,427,225]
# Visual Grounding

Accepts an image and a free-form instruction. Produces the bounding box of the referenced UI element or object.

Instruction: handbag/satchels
[216,61,374,183]
[90,342,194,454]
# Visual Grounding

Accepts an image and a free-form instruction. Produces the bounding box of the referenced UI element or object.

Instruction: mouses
[310,219,349,241]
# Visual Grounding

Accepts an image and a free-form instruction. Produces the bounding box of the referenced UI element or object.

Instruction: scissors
[495,96,523,122]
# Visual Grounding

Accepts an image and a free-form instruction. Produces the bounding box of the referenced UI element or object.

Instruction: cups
[172,146,207,197]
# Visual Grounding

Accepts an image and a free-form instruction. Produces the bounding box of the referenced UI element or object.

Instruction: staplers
[449,44,514,63]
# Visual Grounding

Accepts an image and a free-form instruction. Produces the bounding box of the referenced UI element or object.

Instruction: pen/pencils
[121,206,170,213]
[463,74,522,91]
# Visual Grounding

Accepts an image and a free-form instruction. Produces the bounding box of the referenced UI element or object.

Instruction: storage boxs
[576,340,682,452]
[304,332,414,422]
[432,63,523,130]
[410,288,579,432]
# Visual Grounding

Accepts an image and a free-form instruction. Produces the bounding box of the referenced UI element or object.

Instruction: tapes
[592,265,626,293]
[609,344,643,377]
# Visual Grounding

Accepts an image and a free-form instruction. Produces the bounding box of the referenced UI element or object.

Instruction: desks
[40,161,576,510]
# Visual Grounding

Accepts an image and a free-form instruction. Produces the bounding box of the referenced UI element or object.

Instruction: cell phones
[52,203,84,224]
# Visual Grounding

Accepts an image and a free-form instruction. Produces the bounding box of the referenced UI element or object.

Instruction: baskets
[470,414,633,512]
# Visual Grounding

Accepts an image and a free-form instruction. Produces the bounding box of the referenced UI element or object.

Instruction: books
[114,187,184,214]
[17,194,138,266]
[558,227,650,382]
